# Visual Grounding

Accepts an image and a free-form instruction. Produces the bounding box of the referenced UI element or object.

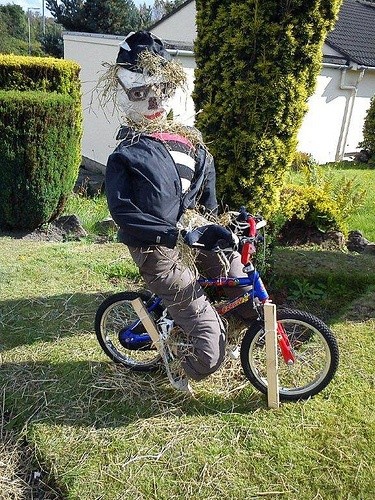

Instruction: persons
[84,29,262,393]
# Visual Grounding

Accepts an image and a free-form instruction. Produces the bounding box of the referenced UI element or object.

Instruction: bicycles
[93,210,339,404]
